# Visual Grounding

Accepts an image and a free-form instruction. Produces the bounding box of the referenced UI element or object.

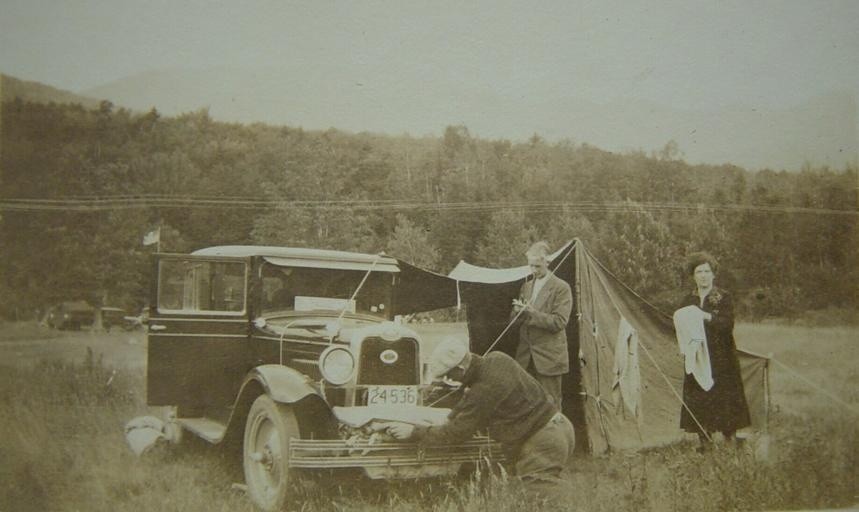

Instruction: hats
[430,335,469,377]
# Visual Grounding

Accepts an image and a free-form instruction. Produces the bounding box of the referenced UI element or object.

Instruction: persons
[387,337,577,487]
[680,249,751,453]
[509,240,573,412]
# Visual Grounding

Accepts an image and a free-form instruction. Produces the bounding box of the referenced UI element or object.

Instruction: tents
[385,235,770,453]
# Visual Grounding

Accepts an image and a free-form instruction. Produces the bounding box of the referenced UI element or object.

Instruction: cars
[145,245,507,509]
[44,300,149,331]
